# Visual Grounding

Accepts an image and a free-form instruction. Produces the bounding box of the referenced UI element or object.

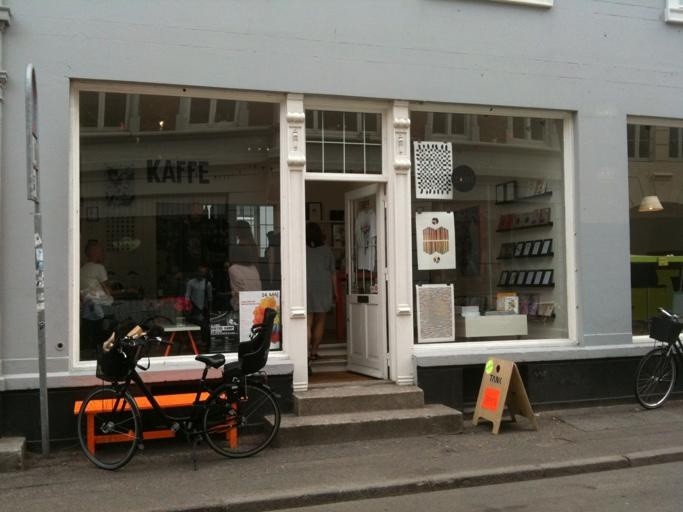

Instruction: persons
[183,214,281,362]
[306,221,342,361]
[80,239,140,343]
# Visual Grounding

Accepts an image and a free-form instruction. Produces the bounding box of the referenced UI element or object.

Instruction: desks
[153,320,202,356]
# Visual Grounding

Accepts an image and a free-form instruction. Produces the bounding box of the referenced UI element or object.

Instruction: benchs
[73,390,252,463]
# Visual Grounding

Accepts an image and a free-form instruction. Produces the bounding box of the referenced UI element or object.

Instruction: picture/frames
[493,179,553,287]
[416,282,456,343]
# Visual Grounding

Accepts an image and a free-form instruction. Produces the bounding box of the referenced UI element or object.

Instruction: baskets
[649,316,683,345]
[93,340,141,383]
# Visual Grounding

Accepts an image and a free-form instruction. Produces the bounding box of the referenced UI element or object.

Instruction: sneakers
[311,354,318,360]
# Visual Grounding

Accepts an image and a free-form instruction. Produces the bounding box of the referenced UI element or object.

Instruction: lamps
[639,176,665,213]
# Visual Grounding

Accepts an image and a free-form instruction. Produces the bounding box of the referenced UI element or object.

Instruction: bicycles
[632,304,683,409]
[77,308,281,471]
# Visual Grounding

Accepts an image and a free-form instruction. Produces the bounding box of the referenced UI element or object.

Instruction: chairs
[223,308,276,403]
